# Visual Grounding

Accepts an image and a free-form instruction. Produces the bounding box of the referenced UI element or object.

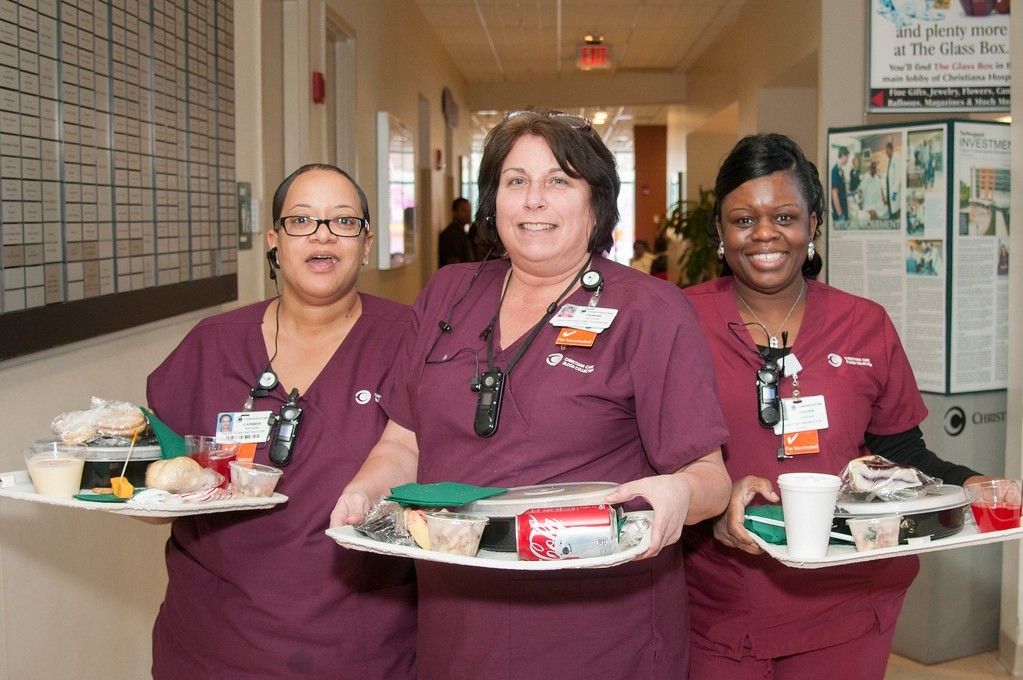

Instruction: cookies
[60,409,153,443]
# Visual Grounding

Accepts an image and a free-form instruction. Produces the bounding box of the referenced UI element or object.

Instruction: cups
[184,434,240,489]
[22,442,88,497]
[963,480,1023,533]
[776,473,842,558]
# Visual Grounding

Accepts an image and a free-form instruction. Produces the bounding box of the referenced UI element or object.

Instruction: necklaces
[735,280,804,349]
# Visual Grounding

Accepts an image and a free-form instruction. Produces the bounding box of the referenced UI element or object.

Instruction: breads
[144,457,210,493]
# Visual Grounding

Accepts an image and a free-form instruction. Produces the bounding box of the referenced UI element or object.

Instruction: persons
[680,135,1022,680]
[907,194,924,230]
[850,143,902,220]
[329,109,730,678]
[650,237,667,274]
[999,244,1008,269]
[631,240,655,275]
[137,164,417,678]
[559,307,574,318]
[219,414,232,432]
[907,240,940,276]
[907,138,935,186]
[833,147,849,221]
[438,197,472,267]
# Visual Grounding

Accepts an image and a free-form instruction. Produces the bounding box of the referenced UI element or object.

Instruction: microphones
[268,259,276,280]
[781,331,788,348]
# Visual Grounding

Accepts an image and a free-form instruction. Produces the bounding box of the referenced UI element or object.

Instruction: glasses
[502,109,593,135]
[274,215,371,238]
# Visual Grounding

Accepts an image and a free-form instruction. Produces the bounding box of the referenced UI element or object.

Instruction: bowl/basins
[424,513,489,557]
[229,461,283,496]
[845,514,903,552]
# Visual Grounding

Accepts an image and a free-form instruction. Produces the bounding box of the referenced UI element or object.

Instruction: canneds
[515,503,619,561]
